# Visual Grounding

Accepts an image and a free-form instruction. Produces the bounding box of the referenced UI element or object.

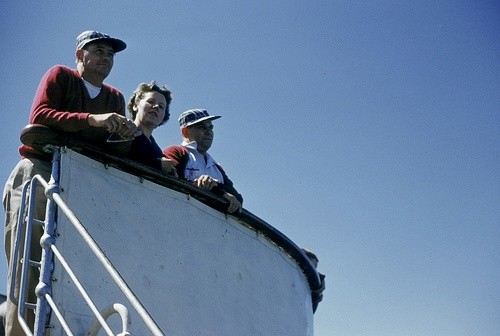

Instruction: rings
[122,121,126,125]
[208,178,212,183]
[168,163,173,168]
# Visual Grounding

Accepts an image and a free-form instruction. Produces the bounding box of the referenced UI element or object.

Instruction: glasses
[107,131,135,142]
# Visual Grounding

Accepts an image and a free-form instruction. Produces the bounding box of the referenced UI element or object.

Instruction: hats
[76,31,127,53]
[178,109,222,128]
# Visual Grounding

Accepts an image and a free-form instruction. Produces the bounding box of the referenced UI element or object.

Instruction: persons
[120,80,178,180]
[164,109,243,214]
[2,30,138,336]
